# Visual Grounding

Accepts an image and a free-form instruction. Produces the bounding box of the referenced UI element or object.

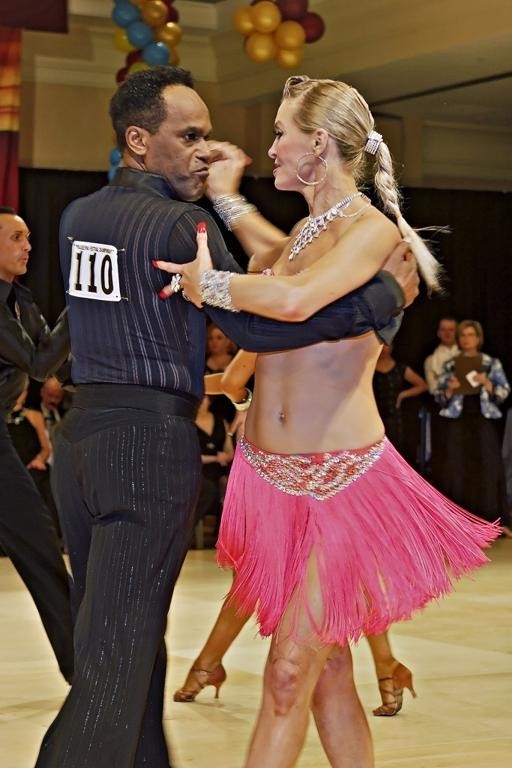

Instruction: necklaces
[288,191,372,262]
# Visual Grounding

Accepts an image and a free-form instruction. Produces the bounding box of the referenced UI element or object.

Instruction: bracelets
[198,268,240,315]
[214,193,253,231]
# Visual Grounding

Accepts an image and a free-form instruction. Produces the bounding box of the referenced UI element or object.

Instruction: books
[233,2,325,69]
[108,1,185,84]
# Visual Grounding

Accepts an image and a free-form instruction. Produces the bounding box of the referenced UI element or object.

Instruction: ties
[48,409,57,425]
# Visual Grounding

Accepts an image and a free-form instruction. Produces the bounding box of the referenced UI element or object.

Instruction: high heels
[374,660,417,717]
[174,663,228,703]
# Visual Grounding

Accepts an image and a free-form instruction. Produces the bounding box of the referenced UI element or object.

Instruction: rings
[182,292,190,301]
[170,272,181,294]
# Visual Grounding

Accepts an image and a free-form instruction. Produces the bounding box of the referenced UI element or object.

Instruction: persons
[33,66,422,767]
[173,140,418,716]
[190,322,255,539]
[152,72,501,766]
[1,205,79,685]
[8,372,67,557]
[373,318,512,540]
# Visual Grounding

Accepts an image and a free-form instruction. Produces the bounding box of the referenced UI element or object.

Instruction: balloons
[107,147,123,180]
